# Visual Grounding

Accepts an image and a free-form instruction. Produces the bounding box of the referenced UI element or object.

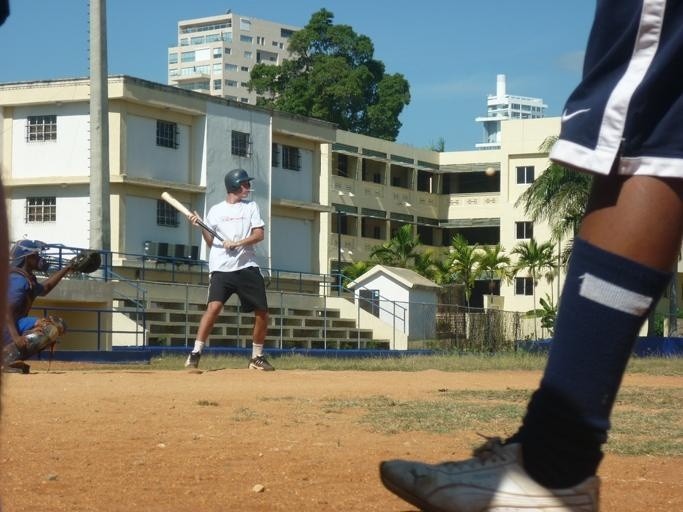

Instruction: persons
[1,238,82,374]
[380,0,680,512]
[184,167,286,372]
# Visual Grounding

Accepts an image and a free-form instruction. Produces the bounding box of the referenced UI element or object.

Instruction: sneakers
[184,352,200,367]
[248,356,275,371]
[0,366,22,373]
[380,433,600,512]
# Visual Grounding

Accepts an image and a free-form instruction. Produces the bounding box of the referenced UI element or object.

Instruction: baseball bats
[160,191,234,250]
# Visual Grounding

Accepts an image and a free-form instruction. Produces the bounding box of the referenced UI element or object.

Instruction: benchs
[111,297,390,350]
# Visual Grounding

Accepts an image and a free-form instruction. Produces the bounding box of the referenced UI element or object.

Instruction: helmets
[9,240,49,272]
[225,169,254,193]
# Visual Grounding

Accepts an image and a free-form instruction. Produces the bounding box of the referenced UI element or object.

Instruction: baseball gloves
[66,250,100,273]
